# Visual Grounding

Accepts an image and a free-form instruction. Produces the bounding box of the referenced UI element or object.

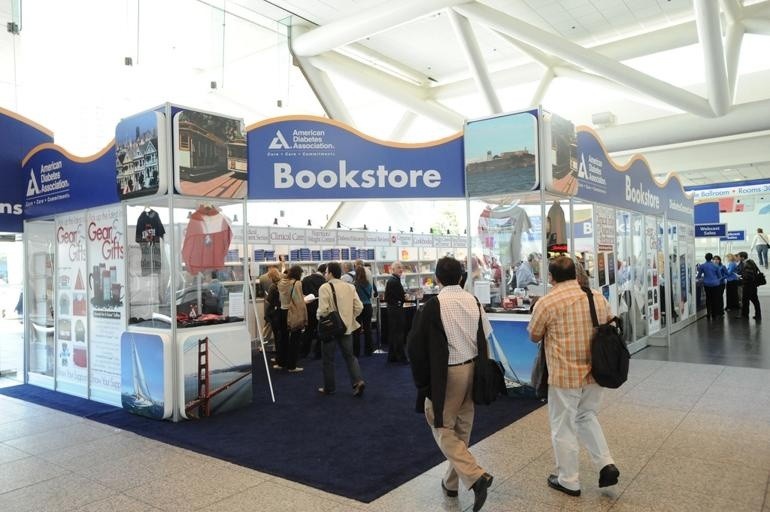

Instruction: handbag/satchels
[472,358,509,406]
[317,311,346,342]
[287,303,306,331]
[756,272,766,285]
[590,317,631,388]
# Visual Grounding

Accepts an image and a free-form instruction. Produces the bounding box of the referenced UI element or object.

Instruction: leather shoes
[472,473,493,512]
[598,463,619,488]
[318,388,335,394]
[353,380,365,398]
[441,479,458,497]
[547,474,581,496]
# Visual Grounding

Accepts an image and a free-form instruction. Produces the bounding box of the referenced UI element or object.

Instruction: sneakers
[289,367,303,372]
[273,364,282,369]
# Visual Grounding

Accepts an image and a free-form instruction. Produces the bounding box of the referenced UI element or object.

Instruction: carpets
[0,318,553,505]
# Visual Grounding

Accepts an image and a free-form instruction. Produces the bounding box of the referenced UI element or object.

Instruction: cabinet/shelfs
[125,223,475,321]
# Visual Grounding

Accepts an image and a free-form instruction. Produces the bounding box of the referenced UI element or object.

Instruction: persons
[258,259,378,398]
[458,251,638,296]
[751,228,770,270]
[697,252,761,321]
[384,262,409,363]
[526,257,620,497]
[205,270,228,315]
[407,256,494,512]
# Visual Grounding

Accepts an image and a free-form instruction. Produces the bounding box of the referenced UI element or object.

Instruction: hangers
[483,205,493,211]
[553,198,561,205]
[495,198,515,209]
[193,199,216,211]
[143,202,153,212]
